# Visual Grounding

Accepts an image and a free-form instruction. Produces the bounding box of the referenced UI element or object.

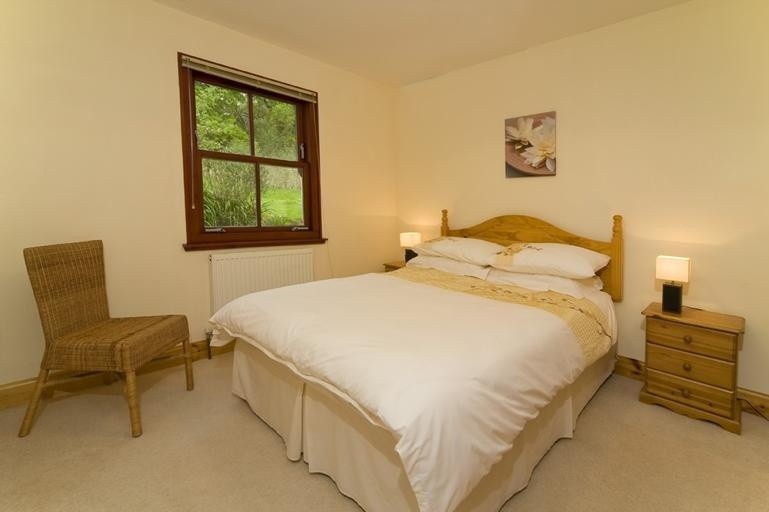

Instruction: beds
[208,205,629,510]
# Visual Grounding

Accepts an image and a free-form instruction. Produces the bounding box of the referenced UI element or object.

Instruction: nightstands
[639,301,745,436]
[382,260,404,274]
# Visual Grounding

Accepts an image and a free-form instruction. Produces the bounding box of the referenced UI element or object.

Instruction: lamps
[399,229,421,261]
[656,255,692,314]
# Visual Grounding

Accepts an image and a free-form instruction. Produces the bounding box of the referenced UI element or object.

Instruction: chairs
[18,238,195,440]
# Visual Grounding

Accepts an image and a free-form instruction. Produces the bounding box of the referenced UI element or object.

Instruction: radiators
[207,247,314,313]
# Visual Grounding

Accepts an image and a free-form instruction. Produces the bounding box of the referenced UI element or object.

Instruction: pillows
[407,255,489,281]
[488,267,598,297]
[492,240,604,274]
[415,236,493,265]
